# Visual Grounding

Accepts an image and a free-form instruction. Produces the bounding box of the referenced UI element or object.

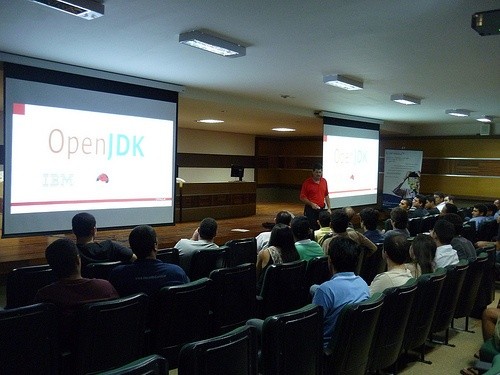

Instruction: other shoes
[474,352,480,358]
[460,367,478,375]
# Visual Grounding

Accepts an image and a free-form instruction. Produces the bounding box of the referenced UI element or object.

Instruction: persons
[106,226,188,298]
[256,192,500,375]
[58,210,137,275]
[173,217,219,256]
[37,238,120,302]
[297,164,331,214]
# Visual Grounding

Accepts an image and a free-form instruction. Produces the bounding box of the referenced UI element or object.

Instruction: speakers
[479,123,491,136]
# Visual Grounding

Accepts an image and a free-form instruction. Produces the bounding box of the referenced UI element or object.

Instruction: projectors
[471,7,500,37]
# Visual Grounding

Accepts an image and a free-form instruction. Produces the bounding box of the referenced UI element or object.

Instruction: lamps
[322,74,364,91]
[476,115,493,122]
[30,0,105,20]
[446,109,471,117]
[391,94,421,105]
[179,31,247,57]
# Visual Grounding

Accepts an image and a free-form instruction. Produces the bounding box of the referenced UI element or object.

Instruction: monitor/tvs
[231,164,244,181]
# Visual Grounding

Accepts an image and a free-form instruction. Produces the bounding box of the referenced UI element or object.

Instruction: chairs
[0,205,500,375]
